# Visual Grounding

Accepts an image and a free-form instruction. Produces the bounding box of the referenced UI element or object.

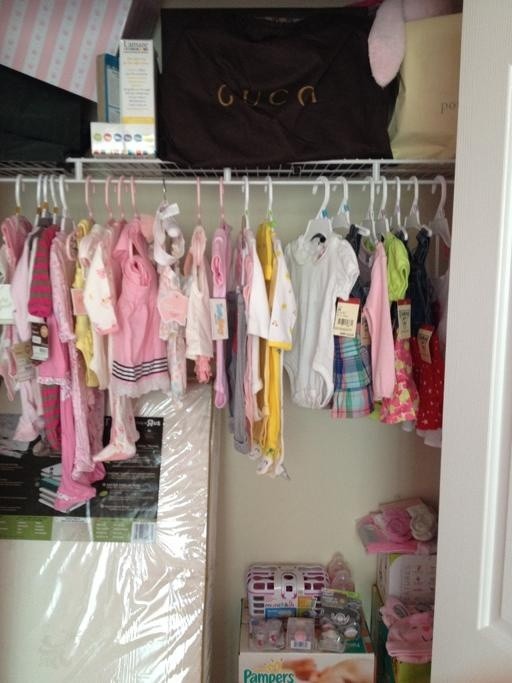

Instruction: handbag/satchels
[159,9,399,171]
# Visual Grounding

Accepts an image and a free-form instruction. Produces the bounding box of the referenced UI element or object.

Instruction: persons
[281,656,374,682]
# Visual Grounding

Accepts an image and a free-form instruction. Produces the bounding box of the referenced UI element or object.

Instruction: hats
[380,601,434,664]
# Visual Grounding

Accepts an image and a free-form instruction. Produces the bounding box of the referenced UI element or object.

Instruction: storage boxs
[238,551,436,683]
